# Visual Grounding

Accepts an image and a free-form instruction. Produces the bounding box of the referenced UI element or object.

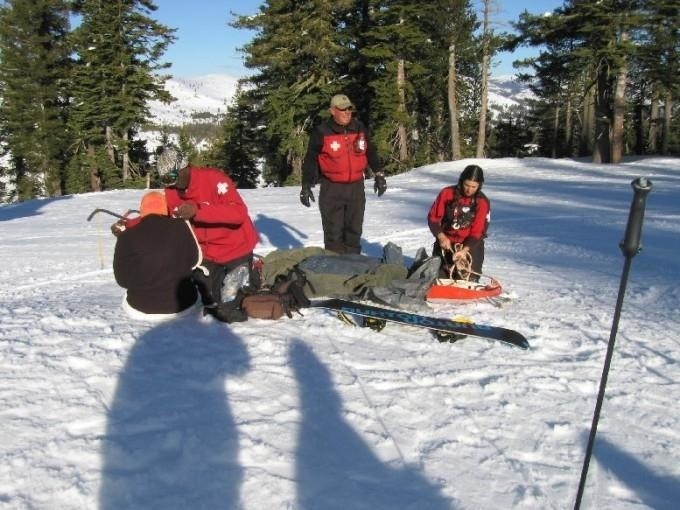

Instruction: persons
[299,93,387,256]
[156,147,263,293]
[428,165,491,285]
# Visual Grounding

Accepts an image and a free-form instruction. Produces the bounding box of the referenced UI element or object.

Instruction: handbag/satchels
[344,243,441,311]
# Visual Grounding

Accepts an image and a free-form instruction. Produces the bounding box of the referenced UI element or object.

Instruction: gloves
[111,221,127,237]
[171,204,196,218]
[373,176,387,197]
[300,184,315,207]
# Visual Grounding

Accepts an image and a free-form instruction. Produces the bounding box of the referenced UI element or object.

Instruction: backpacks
[242,294,285,319]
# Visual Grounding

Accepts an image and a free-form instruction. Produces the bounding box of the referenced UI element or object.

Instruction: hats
[330,94,355,110]
[141,192,168,217]
[157,150,190,177]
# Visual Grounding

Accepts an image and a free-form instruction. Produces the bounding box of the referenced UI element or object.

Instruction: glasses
[340,107,352,111]
[161,173,179,187]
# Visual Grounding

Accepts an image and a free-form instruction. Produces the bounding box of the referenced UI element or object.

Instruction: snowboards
[312,299,530,350]
